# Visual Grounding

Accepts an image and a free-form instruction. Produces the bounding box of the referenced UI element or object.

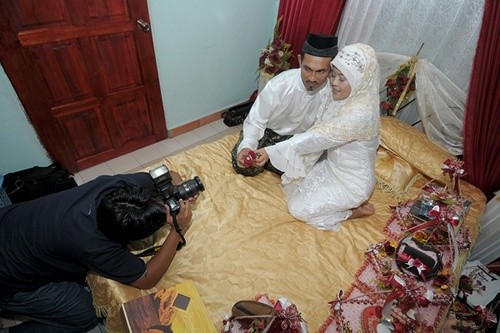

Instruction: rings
[246,157,250,161]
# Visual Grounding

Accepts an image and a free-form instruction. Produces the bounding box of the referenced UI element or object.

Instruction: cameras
[149,165,205,216]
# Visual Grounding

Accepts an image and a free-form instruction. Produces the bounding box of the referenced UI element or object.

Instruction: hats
[302,33,338,57]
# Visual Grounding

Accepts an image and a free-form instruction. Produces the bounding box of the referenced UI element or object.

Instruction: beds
[84,131,487,333]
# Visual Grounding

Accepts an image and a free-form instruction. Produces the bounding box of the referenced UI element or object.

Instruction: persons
[0,171,200,333]
[252,43,380,231]
[231,33,338,177]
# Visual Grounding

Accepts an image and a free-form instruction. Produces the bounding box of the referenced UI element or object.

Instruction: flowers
[379,55,420,116]
[253,15,296,81]
[243,150,257,168]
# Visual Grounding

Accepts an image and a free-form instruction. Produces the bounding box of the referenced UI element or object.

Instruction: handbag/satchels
[221,103,253,127]
[2,161,78,204]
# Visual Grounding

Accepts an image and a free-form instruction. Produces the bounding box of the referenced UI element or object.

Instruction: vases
[258,72,274,96]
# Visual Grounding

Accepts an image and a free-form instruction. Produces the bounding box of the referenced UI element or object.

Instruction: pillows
[375,146,424,202]
[379,116,457,186]
